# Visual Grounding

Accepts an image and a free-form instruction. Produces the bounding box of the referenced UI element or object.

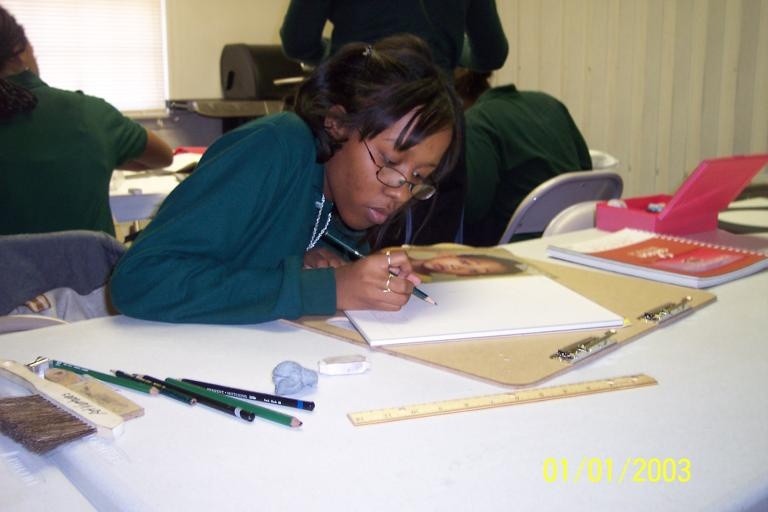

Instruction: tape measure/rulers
[348,373,658,426]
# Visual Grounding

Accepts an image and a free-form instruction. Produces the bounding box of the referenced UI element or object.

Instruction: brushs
[0,359,124,454]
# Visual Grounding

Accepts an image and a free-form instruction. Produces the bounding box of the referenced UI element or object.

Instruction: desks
[109,170,184,234]
[0,227,768,512]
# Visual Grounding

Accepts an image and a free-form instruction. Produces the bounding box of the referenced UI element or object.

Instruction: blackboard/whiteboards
[0,2,172,121]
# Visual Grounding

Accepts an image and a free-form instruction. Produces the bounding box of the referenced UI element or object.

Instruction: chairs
[495,170,624,246]
[541,199,628,240]
[0,314,69,333]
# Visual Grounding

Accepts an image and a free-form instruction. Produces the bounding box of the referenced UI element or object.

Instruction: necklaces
[305,196,334,248]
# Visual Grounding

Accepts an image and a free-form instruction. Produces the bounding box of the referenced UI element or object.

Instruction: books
[542,224,767,289]
[341,272,629,347]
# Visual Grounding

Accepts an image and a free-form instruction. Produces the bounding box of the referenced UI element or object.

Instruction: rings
[386,275,392,292]
[385,248,392,267]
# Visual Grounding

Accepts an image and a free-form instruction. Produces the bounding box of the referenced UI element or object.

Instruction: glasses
[356,126,439,202]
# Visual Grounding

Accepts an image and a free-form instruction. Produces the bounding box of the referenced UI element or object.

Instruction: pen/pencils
[38,357,314,427]
[325,233,438,306]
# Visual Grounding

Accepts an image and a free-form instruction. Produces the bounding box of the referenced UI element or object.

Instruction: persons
[455,68,593,247]
[109,34,470,327]
[404,252,528,287]
[1,3,172,239]
[281,0,510,247]
